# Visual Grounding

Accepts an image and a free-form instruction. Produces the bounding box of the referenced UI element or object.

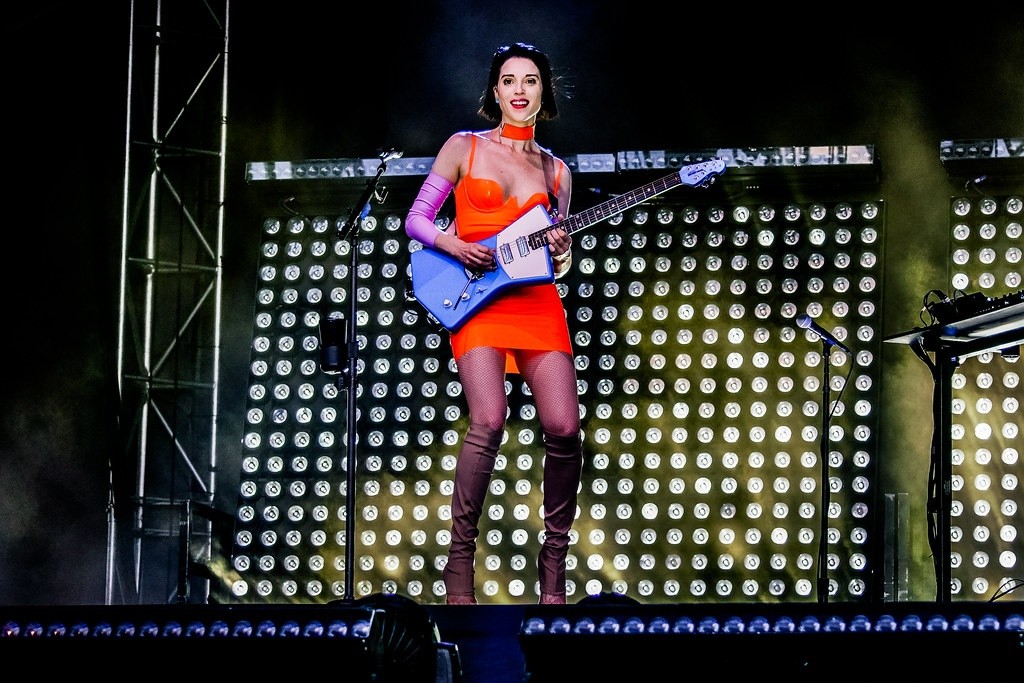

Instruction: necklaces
[498,123,535,144]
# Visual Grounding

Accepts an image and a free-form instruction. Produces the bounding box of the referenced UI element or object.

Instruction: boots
[537,430,584,604]
[442,422,504,605]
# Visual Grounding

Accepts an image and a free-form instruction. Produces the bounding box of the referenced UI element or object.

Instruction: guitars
[411,157,727,335]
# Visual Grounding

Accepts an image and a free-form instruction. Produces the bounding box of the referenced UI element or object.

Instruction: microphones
[796,313,851,354]
[380,147,405,158]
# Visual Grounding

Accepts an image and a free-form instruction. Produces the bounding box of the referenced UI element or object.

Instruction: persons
[404,42,583,606]
